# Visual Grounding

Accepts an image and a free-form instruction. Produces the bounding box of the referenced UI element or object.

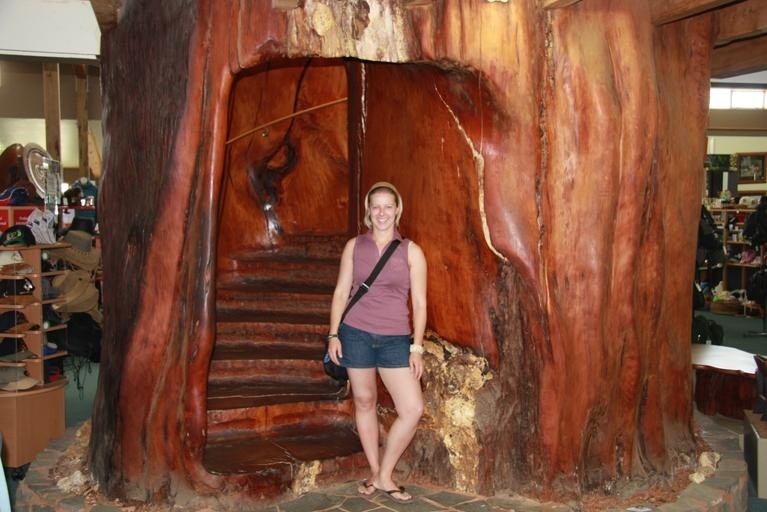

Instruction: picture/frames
[736,151,767,185]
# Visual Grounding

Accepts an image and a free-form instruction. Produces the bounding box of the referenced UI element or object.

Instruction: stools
[690,342,759,416]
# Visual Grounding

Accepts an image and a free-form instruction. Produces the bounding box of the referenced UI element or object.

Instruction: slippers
[376,487,414,505]
[358,483,374,498]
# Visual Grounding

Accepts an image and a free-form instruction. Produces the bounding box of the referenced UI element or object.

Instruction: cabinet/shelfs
[692,208,764,318]
[0,242,70,481]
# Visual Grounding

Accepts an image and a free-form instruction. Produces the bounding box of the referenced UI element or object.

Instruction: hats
[1,224,36,246]
[0,279,42,305]
[52,217,99,313]
[0,311,41,334]
[1,367,40,392]
[0,250,33,275]
[0,338,38,361]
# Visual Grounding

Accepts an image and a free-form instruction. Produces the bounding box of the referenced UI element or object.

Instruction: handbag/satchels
[322,331,348,381]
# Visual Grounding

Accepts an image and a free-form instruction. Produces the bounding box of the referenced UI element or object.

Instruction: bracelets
[409,344,423,354]
[327,334,337,339]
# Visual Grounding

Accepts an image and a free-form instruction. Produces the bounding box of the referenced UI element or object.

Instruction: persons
[327,181,426,503]
